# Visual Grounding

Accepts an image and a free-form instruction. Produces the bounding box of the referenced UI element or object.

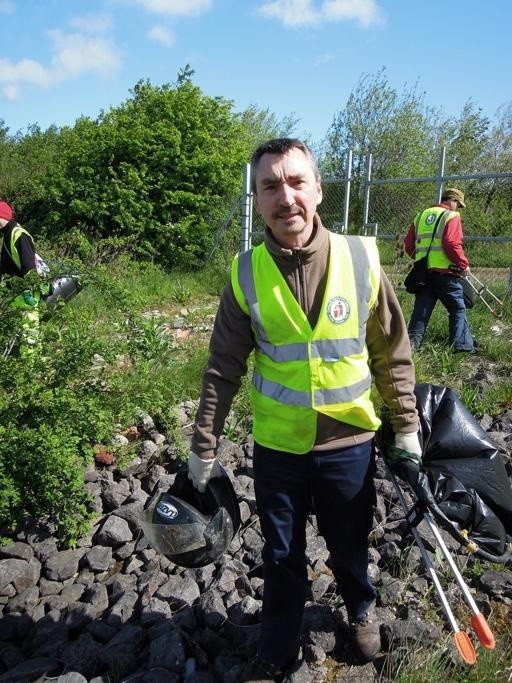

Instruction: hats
[0,200,15,222]
[443,188,466,208]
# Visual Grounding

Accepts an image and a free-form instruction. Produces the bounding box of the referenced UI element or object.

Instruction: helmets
[139,461,241,569]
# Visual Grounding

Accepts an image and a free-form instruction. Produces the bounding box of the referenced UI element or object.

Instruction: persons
[184,136,425,683]
[402,187,477,355]
[1,198,49,366]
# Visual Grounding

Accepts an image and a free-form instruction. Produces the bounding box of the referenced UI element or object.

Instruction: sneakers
[347,602,382,663]
[235,633,301,683]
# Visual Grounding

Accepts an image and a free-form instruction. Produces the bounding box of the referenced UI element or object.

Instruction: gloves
[186,449,223,493]
[22,291,37,305]
[395,429,423,459]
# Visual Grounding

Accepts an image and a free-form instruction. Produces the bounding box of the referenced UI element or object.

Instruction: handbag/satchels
[404,255,429,293]
